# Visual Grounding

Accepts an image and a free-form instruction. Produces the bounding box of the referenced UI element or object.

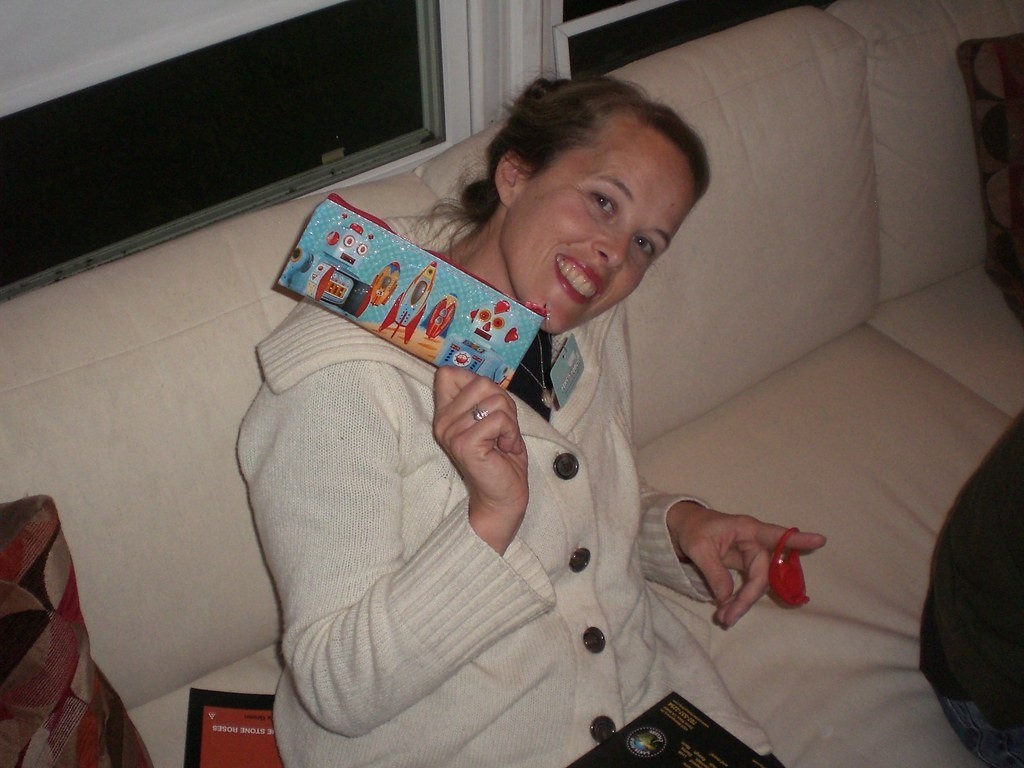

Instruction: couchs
[0,0,1024,768]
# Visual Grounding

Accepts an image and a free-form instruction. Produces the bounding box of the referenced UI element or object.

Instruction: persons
[237,71,826,768]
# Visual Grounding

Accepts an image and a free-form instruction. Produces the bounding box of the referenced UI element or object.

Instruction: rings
[769,551,784,565]
[473,405,489,421]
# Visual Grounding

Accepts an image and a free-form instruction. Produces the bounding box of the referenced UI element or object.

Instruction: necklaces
[520,332,551,408]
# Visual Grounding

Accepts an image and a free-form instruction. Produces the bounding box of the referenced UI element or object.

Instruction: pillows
[0,495,153,768]
[958,34,1023,323]
